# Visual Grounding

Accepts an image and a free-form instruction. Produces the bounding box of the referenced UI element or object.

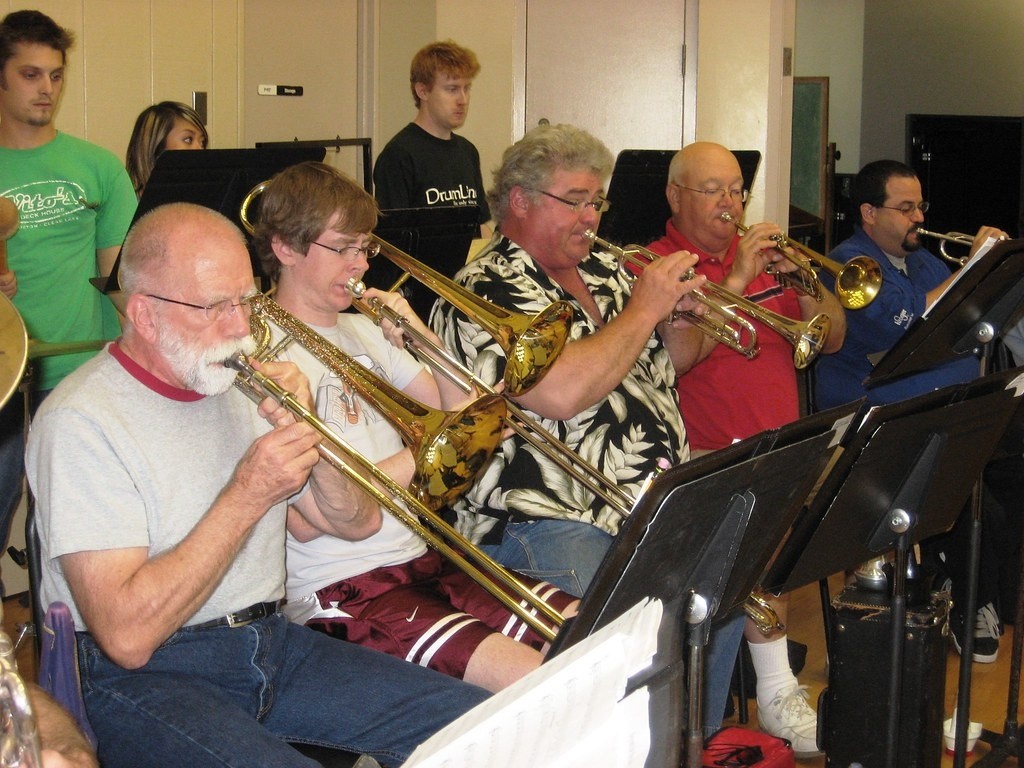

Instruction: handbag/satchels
[702,726,796,768]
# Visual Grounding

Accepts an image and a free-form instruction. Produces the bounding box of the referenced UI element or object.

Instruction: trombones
[219,286,565,645]
[237,178,784,644]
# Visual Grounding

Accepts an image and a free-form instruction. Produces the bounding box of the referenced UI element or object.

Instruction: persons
[125,102,209,204]
[363,38,495,332]
[23,124,1024,768]
[0,9,141,595]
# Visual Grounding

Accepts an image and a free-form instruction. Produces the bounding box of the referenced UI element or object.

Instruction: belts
[81,600,288,635]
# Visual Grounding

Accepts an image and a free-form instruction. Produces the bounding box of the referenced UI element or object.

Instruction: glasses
[881,201,930,216]
[714,745,763,767]
[310,240,382,261]
[676,184,751,202]
[515,184,611,212]
[145,291,264,321]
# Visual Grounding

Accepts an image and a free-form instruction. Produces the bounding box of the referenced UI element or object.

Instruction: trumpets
[915,225,1011,270]
[1,600,47,768]
[581,227,833,371]
[718,211,884,310]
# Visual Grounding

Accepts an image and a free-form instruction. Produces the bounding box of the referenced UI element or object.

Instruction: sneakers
[951,603,1000,663]
[756,682,826,759]
[825,654,830,676]
